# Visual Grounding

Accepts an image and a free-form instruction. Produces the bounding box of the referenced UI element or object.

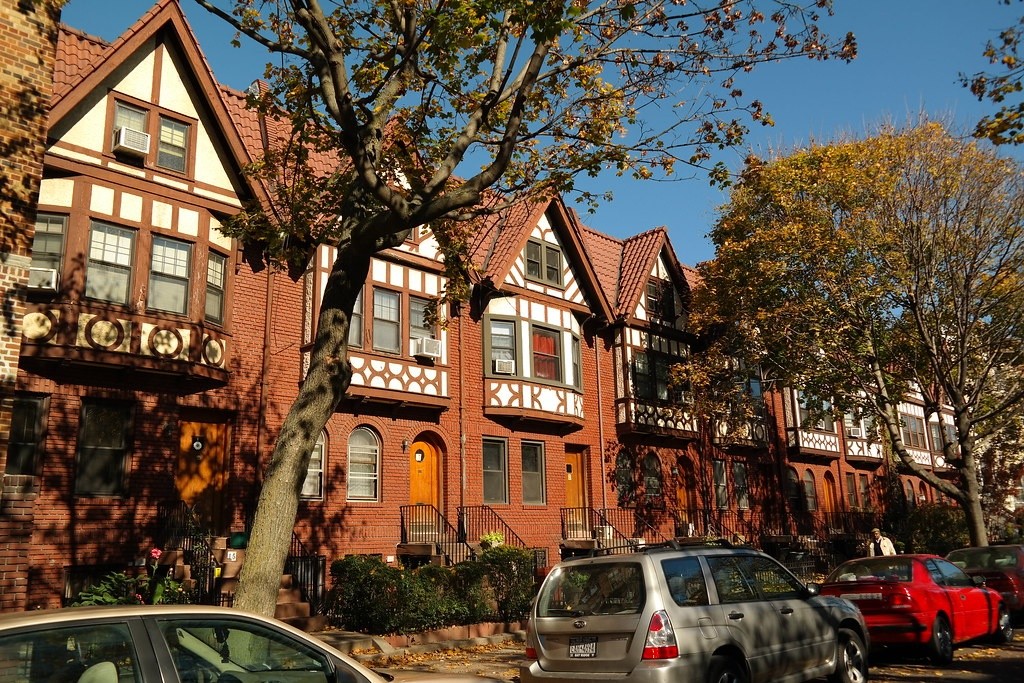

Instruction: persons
[866,528,896,556]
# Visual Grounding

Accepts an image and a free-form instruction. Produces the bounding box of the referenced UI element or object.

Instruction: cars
[0,604,517,683]
[945,544,1024,614]
[816,554,1015,663]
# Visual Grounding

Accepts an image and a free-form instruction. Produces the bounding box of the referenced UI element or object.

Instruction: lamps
[401,437,407,454]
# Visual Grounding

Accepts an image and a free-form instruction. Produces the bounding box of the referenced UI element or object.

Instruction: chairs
[855,565,872,576]
[667,575,685,603]
[952,562,967,569]
[49,659,119,683]
[713,569,730,598]
[995,559,1009,566]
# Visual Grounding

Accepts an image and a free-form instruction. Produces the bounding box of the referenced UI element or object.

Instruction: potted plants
[479,529,504,550]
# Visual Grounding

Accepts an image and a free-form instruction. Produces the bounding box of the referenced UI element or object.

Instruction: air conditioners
[112,126,151,158]
[414,337,441,358]
[815,420,825,429]
[676,391,694,404]
[28,267,58,292]
[493,359,515,373]
[847,428,861,437]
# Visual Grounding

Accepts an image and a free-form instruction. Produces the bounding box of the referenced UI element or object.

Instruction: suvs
[516,533,870,683]
